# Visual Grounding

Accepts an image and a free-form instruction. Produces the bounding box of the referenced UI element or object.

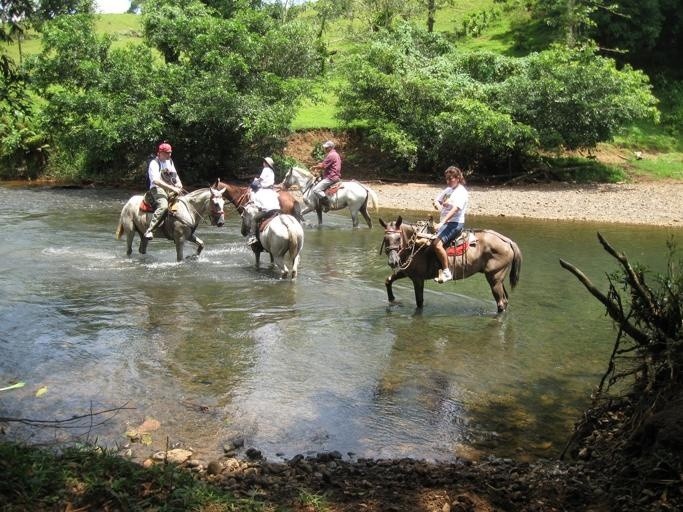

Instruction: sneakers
[322,201,330,213]
[436,271,453,282]
[143,232,153,239]
[246,237,258,246]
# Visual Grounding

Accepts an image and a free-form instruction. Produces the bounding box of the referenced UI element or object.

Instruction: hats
[263,156,275,169]
[251,180,262,190]
[158,144,172,153]
[322,140,335,149]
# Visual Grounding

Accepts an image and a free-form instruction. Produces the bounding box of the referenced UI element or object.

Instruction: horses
[115,185,227,262]
[378,215,522,326]
[280,165,379,228]
[239,204,304,279]
[212,177,301,223]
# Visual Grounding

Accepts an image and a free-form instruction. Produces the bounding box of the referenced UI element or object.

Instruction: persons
[141,144,183,238]
[245,179,282,246]
[431,166,468,285]
[310,138,342,213]
[247,155,276,203]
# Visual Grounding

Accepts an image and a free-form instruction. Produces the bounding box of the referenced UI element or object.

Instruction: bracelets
[441,220,446,224]
